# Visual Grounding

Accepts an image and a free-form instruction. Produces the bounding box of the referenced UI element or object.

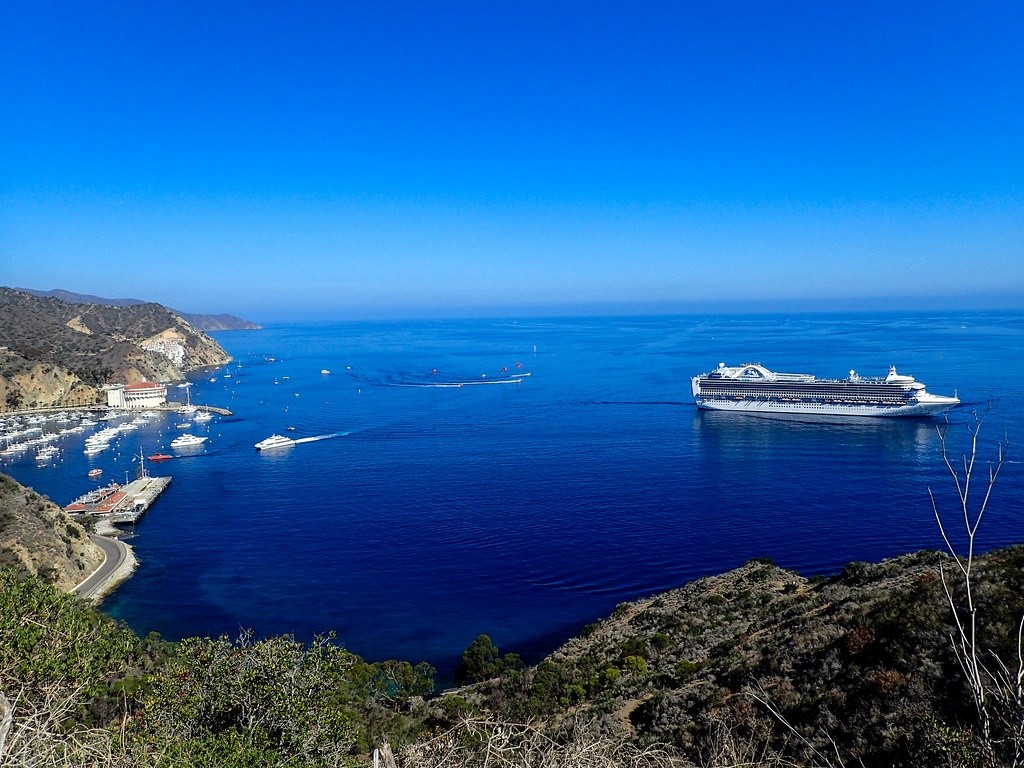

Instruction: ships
[690,362,960,416]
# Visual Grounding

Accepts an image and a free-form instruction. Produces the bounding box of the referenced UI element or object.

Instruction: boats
[170,433,208,447]
[253,436,297,451]
[88,468,102,477]
[177,422,191,430]
[287,426,295,431]
[0,405,158,468]
[147,453,172,460]
[210,379,216,382]
[321,370,330,374]
[194,410,213,420]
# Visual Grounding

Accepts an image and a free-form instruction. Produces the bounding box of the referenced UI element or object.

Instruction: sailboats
[177,384,198,414]
[224,368,232,378]
[237,360,242,367]
